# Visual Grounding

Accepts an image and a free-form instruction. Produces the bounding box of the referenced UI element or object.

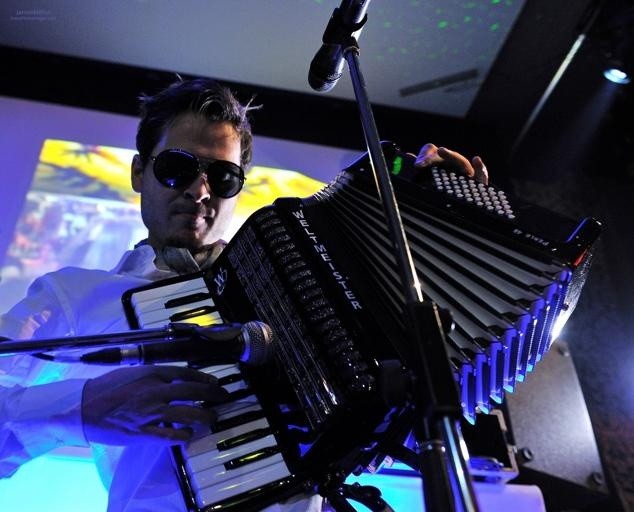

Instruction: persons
[1,73,488,512]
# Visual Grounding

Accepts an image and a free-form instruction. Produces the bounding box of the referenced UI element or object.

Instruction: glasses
[142,148,248,200]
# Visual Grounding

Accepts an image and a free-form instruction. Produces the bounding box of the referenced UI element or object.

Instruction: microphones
[308,1,371,93]
[81,320,274,366]
